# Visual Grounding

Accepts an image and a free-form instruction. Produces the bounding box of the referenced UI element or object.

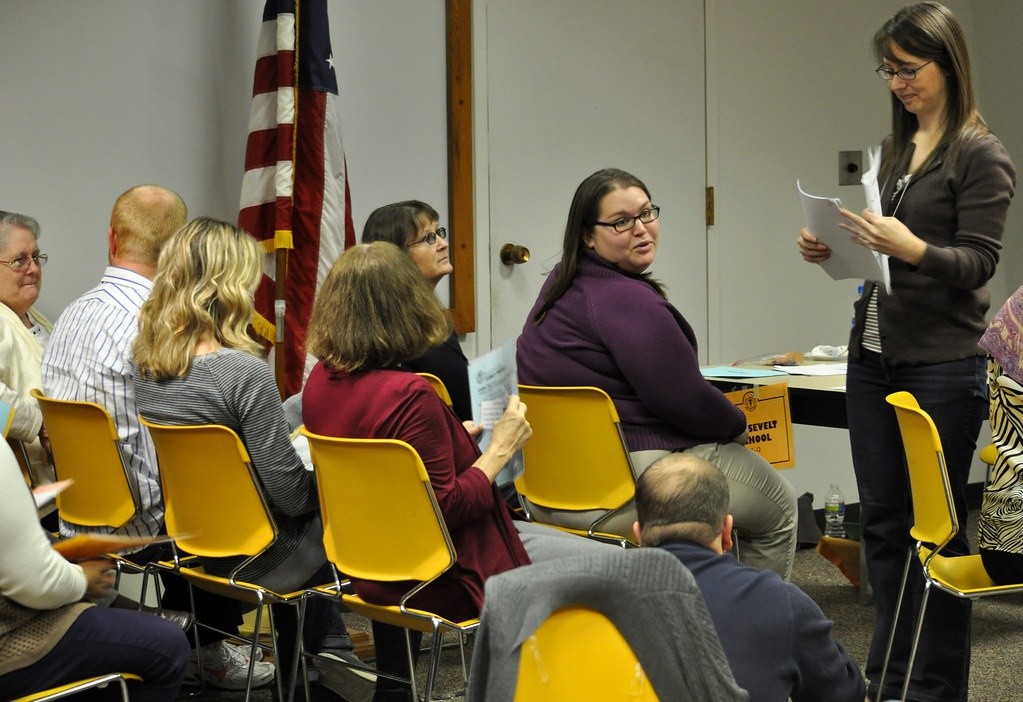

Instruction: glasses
[594,203,660,233]
[875,61,935,80]
[407,225,447,245]
[0,253,48,271]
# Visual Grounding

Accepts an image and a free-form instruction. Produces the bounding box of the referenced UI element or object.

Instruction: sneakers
[234,645,263,662]
[314,652,376,702]
[185,642,273,687]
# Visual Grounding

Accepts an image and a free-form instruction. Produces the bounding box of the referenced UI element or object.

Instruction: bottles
[825,483,846,539]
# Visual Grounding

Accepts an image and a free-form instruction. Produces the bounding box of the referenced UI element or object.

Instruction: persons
[514,170,798,581]
[634,453,870,702]
[362,201,471,427]
[302,243,626,626]
[0,209,68,485]
[0,431,191,702]
[797,0,1017,702]
[40,186,275,690]
[976,285,1023,585]
[131,216,377,701]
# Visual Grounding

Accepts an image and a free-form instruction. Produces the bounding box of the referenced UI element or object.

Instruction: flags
[239,1,356,403]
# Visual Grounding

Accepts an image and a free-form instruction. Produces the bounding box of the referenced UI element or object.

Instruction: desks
[702,354,990,563]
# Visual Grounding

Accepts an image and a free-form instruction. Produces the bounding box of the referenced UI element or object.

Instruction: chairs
[0,370,1023,702]
